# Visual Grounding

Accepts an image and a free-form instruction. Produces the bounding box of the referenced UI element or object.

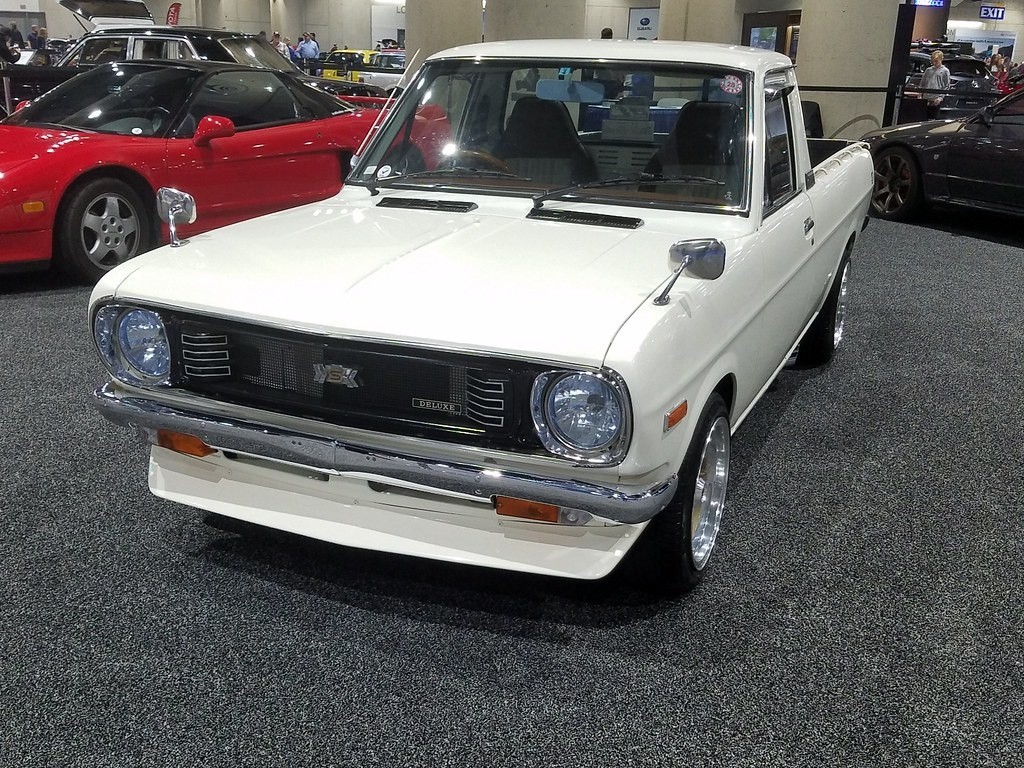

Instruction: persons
[0,21,48,65]
[916,49,951,120]
[592,27,625,99]
[977,44,1018,79]
[372,43,383,52]
[626,35,660,100]
[258,30,321,61]
[331,43,350,54]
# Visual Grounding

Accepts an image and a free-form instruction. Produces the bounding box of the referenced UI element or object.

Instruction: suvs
[906,35,1024,109]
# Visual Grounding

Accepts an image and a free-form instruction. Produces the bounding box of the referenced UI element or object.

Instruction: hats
[273,31,280,37]
[302,32,310,37]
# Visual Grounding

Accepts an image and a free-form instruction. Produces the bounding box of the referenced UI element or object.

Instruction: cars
[23,1,389,107]
[357,58,405,98]
[323,49,380,83]
[858,87,1024,214]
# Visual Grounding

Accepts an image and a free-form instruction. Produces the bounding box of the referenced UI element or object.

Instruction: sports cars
[0,54,451,288]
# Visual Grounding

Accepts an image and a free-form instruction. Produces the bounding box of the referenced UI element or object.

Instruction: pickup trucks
[86,39,876,597]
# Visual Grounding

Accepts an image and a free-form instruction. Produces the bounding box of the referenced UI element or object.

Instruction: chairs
[486,96,599,185]
[638,101,745,205]
